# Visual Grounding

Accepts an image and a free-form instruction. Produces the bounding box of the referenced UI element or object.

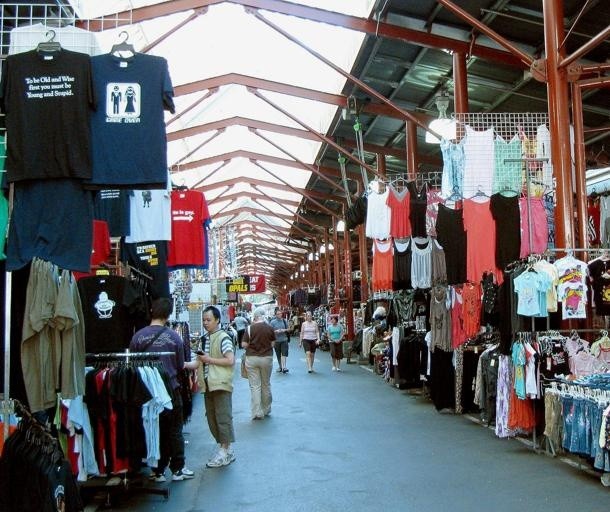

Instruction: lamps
[424,77,456,144]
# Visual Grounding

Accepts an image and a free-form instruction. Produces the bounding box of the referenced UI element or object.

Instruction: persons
[229,310,248,349]
[241,306,276,421]
[242,309,307,337]
[183,305,237,468]
[326,315,346,372]
[269,309,290,374]
[298,311,320,373]
[129,296,194,482]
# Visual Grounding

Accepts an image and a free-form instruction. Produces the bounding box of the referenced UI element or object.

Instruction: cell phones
[196,350,204,355]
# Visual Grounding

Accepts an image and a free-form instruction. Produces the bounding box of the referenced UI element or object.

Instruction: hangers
[176,179,188,191]
[35,30,62,53]
[365,176,519,212]
[516,331,610,404]
[32,256,71,286]
[94,262,149,288]
[13,403,63,475]
[42,7,65,28]
[511,254,608,283]
[93,352,162,371]
[111,31,135,56]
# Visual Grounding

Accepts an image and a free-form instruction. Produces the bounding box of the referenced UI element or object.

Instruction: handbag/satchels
[286,332,290,343]
[241,351,249,379]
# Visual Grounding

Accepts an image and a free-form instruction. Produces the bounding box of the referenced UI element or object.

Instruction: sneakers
[251,411,272,421]
[172,465,196,482]
[275,365,342,373]
[206,446,237,469]
[147,472,167,482]
